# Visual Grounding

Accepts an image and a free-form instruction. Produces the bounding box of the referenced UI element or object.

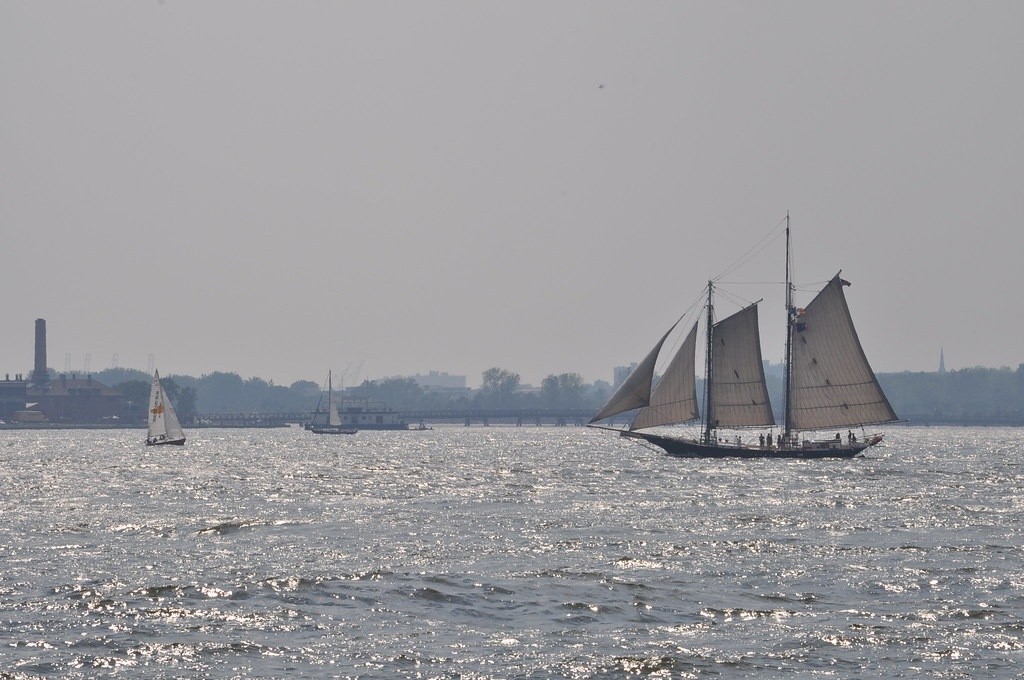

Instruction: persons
[734,435,742,448]
[836,430,856,446]
[759,434,785,451]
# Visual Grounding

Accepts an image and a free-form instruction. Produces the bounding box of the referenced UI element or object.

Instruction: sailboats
[584,209,910,460]
[142,369,186,445]
[307,369,359,434]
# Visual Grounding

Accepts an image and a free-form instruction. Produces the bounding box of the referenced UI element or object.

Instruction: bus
[15,411,44,423]
